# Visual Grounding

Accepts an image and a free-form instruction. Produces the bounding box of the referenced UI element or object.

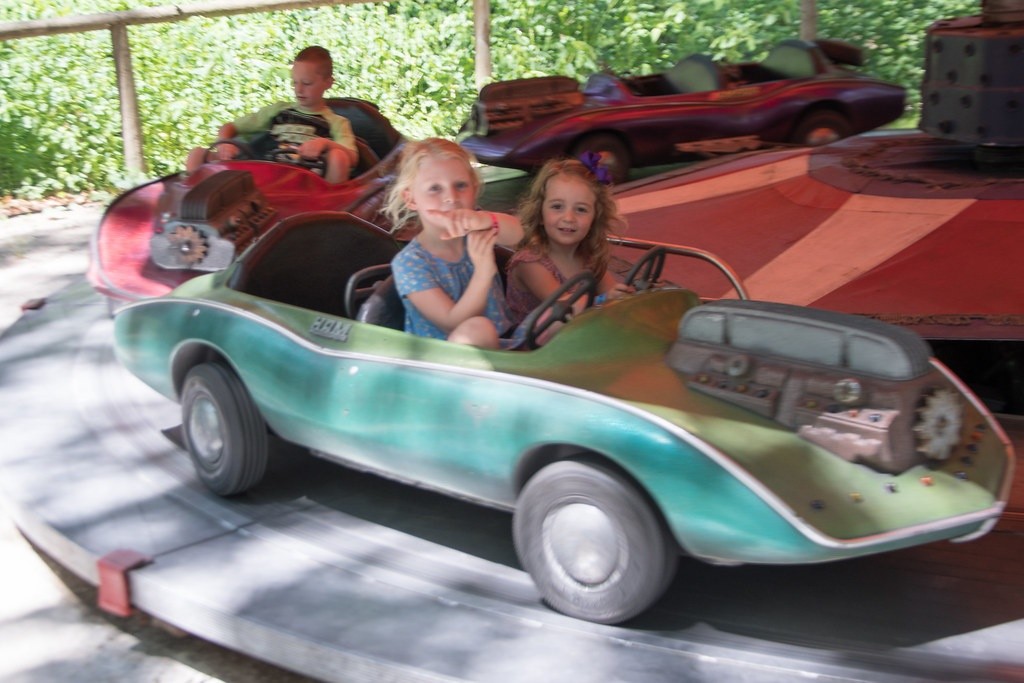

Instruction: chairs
[360,240,516,337]
[302,97,400,161]
[231,206,396,317]
[669,54,721,94]
[761,37,818,83]
[344,135,378,179]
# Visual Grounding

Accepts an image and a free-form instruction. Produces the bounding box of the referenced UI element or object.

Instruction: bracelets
[478,209,501,237]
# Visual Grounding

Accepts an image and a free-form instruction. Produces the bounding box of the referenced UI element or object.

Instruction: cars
[457,35,910,186]
[109,207,1017,624]
[87,96,407,305]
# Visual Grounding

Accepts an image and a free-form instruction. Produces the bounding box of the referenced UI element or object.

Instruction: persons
[378,135,526,351]
[502,157,667,350]
[217,45,361,184]
